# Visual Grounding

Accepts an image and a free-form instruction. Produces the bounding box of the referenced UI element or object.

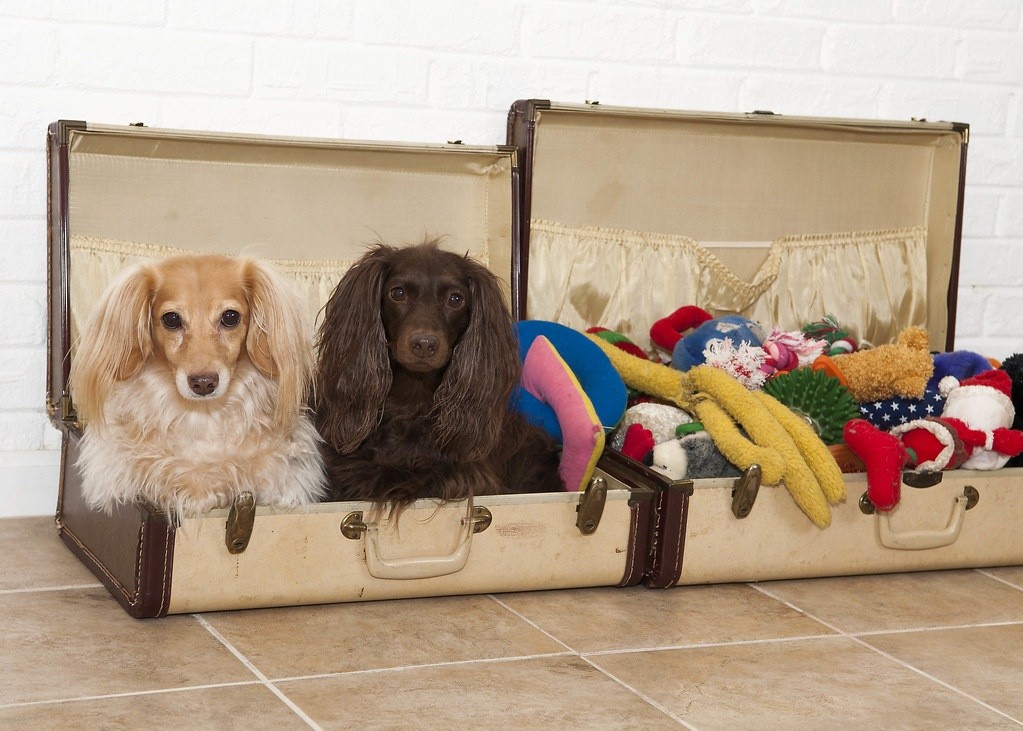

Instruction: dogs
[299,224,567,539]
[61,254,329,539]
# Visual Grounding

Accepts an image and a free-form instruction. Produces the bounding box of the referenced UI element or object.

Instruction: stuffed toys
[505,305,1023,529]
